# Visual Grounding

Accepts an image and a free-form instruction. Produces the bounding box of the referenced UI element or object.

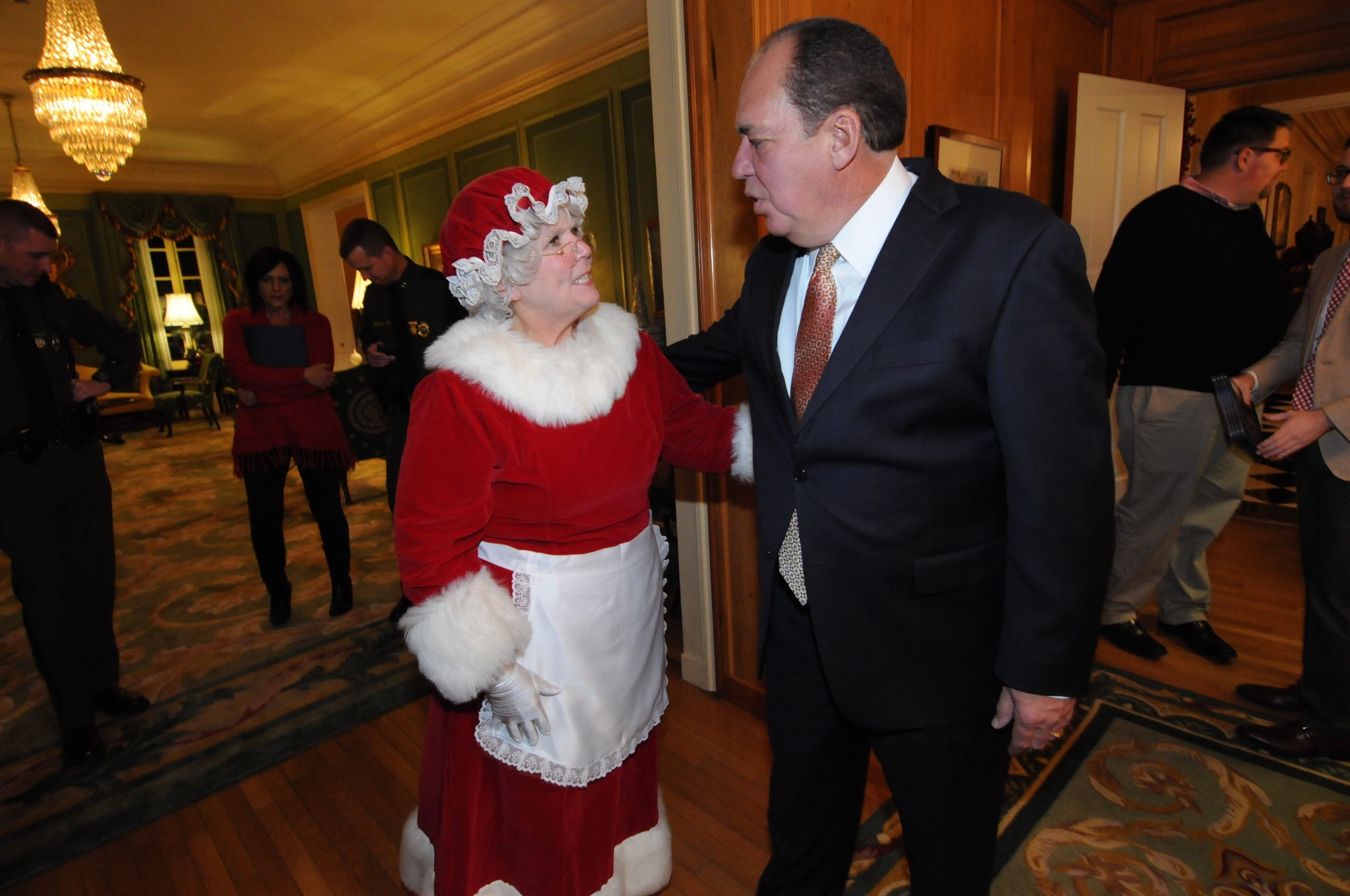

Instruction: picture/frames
[1256,196,1270,230]
[1271,181,1293,250]
[644,219,664,317]
[925,124,1012,192]
[421,241,444,274]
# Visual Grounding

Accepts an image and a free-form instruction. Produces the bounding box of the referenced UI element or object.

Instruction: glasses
[1232,146,1291,166]
[529,232,597,268]
[1326,166,1350,186]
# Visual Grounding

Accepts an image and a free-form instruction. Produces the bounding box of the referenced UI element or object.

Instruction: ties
[1291,249,1350,411]
[790,244,841,426]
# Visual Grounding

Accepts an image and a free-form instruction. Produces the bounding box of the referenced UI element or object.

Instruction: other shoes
[269,580,293,627]
[1156,613,1238,665]
[60,719,107,761]
[1102,619,1167,661]
[96,687,150,715]
[327,577,354,616]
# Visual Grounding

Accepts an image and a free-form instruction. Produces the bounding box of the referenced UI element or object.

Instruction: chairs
[153,353,222,438]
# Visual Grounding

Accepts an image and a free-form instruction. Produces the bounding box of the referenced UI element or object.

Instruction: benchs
[76,362,161,446]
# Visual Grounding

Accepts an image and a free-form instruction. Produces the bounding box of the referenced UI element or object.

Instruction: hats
[441,167,588,310]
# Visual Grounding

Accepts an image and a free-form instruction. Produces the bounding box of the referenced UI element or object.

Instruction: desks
[73,378,111,405]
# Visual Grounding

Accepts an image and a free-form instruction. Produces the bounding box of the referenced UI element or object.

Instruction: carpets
[841,659,1350,896]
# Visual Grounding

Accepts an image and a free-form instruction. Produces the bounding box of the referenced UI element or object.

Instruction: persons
[340,217,469,622]
[222,248,354,628]
[0,199,152,768]
[1232,139,1350,758]
[1097,105,1295,662]
[659,16,1116,896]
[392,169,755,896]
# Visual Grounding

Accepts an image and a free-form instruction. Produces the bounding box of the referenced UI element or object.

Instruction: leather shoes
[1236,716,1350,758]
[1234,673,1307,711]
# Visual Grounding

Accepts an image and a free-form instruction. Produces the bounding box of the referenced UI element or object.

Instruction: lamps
[21,0,148,183]
[10,166,62,237]
[162,292,205,357]
[351,269,373,317]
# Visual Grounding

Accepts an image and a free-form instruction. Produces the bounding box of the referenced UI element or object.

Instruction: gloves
[487,661,561,746]
